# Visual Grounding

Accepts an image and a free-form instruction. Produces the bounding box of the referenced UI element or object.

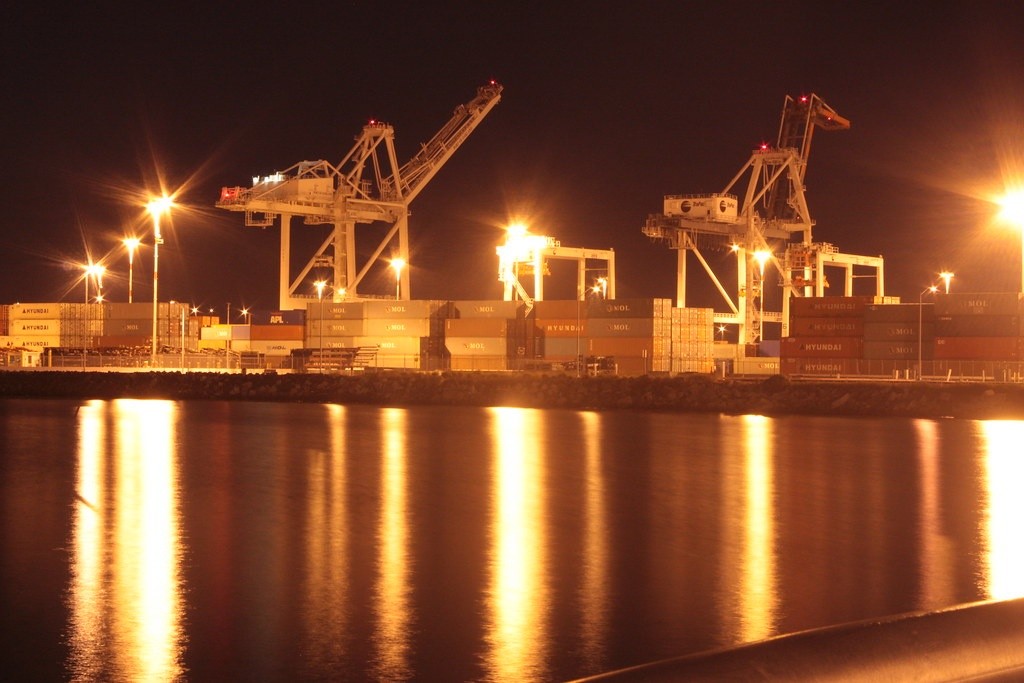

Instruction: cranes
[218,72,507,312]
[640,91,905,349]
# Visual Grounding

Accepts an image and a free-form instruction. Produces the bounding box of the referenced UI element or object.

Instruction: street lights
[123,237,139,303]
[80,261,104,304]
[913,284,942,382]
[390,255,407,300]
[752,250,770,341]
[141,196,180,367]
